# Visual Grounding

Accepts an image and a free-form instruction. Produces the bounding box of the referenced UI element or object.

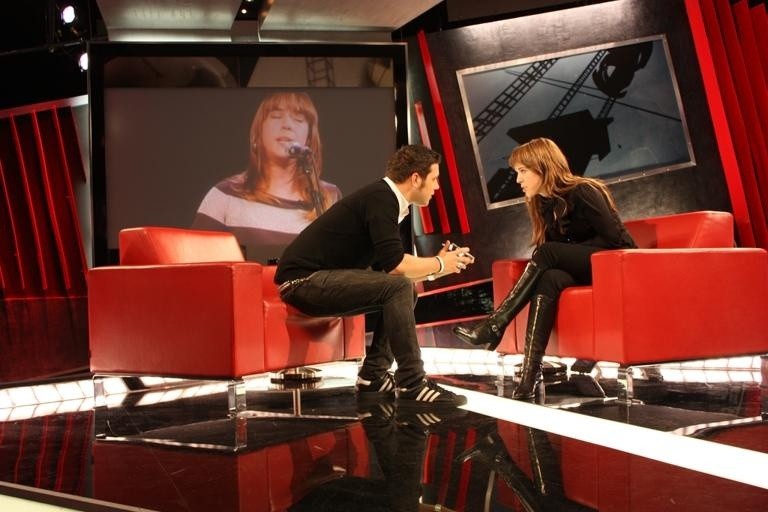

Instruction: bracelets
[435,255,444,274]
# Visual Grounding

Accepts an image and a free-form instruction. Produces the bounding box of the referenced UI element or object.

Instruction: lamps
[60,5,77,24]
[75,49,89,71]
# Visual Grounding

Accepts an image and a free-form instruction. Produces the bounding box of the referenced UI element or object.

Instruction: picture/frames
[454,33,697,210]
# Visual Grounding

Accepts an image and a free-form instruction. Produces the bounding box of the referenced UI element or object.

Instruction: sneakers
[354,373,395,396]
[395,378,467,409]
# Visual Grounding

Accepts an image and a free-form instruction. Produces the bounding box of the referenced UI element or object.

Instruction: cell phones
[441,240,476,265]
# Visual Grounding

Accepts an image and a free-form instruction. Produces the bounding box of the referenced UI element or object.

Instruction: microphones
[284,141,311,158]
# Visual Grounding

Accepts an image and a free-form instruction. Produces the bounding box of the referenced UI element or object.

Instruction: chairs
[496,387,768,511]
[88,389,372,512]
[84,225,368,416]
[489,209,767,404]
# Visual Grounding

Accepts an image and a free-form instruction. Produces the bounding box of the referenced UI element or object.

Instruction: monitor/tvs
[86,42,413,270]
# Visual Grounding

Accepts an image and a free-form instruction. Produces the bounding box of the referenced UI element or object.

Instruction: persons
[450,138,638,399]
[275,144,475,407]
[286,400,470,511]
[452,418,598,511]
[188,87,343,270]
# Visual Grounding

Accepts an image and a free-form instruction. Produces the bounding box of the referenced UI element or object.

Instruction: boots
[513,295,555,399]
[452,261,545,351]
